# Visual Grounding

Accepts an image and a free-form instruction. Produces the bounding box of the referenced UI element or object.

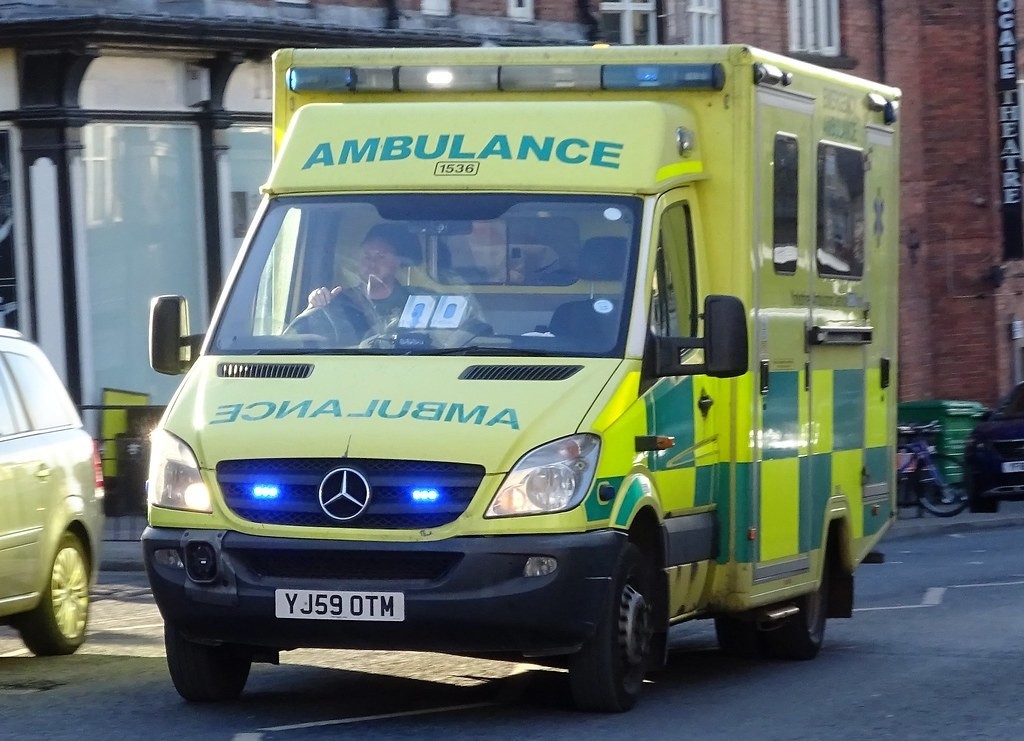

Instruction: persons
[306,236,437,336]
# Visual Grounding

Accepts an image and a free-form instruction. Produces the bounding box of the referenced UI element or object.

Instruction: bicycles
[898,419,971,517]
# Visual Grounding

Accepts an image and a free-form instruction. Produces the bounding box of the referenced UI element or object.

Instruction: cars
[964,382,1024,514]
[0,329,108,657]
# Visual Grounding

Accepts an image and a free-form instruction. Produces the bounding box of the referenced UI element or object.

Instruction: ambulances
[138,43,898,715]
